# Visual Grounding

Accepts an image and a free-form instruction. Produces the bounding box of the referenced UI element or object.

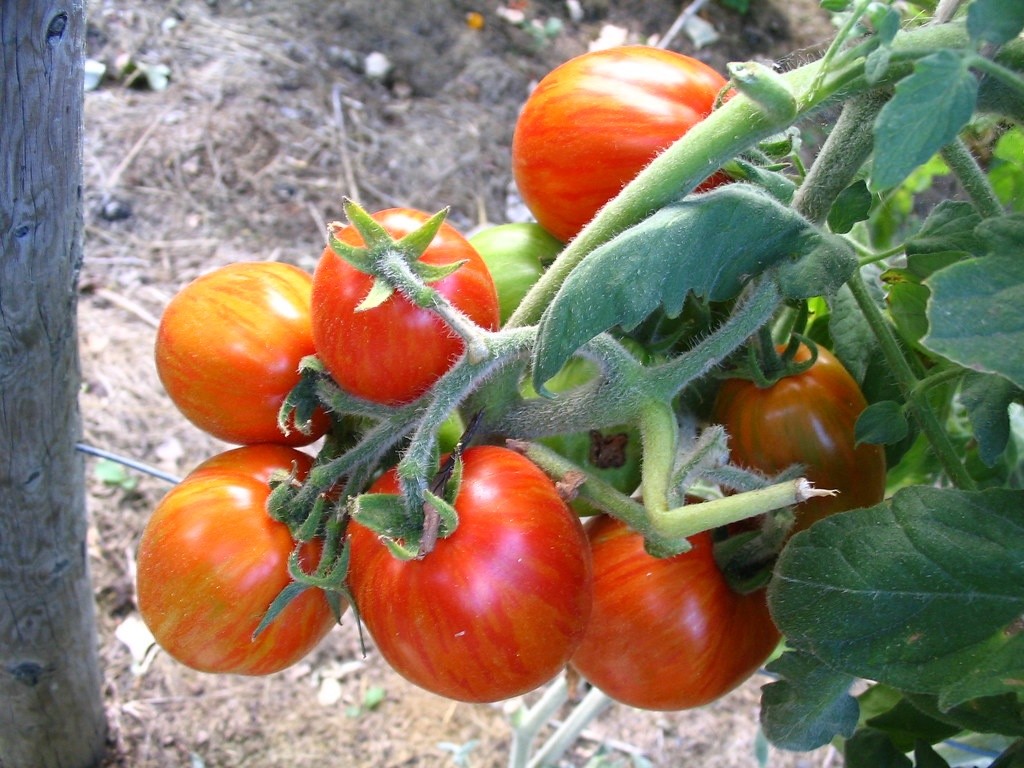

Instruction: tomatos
[137,44,889,712]
